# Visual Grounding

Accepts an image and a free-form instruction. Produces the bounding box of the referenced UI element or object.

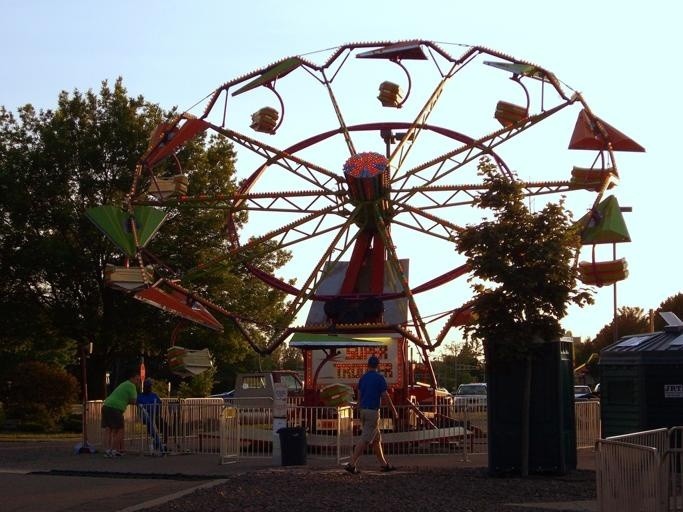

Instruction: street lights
[80,341,93,448]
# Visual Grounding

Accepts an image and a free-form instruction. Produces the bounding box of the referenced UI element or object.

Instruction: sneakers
[104,450,129,458]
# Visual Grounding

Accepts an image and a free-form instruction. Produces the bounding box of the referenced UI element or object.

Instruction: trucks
[234,371,304,407]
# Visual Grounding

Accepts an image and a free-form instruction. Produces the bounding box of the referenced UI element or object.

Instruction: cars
[574,384,600,402]
[206,388,234,403]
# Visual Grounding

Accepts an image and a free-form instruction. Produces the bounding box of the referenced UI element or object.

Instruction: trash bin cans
[275,428,309,466]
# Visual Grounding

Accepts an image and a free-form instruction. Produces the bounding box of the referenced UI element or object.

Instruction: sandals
[343,464,361,474]
[379,463,396,472]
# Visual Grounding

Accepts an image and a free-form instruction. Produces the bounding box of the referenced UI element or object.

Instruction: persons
[100,372,140,458]
[345,357,399,472]
[136,379,166,456]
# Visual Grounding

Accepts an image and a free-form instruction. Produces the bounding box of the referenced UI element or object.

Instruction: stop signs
[141,364,145,382]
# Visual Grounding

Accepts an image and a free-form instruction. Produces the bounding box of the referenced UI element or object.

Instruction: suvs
[454,382,487,412]
